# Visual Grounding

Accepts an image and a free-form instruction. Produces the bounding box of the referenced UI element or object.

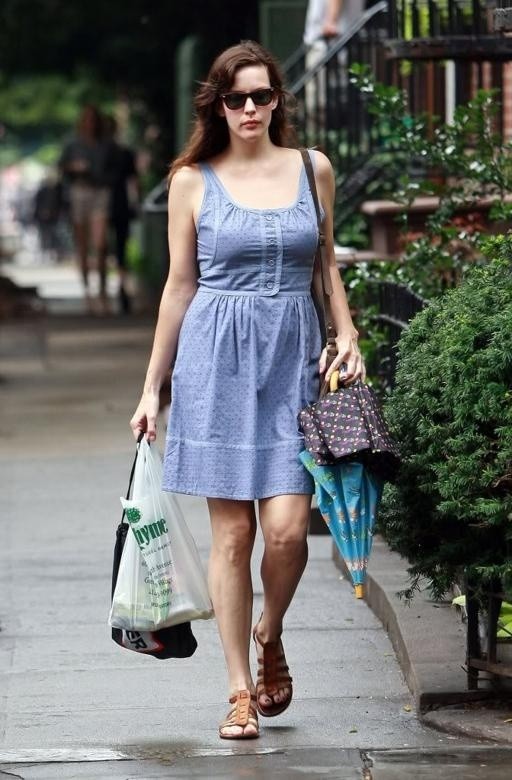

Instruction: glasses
[218,87,276,110]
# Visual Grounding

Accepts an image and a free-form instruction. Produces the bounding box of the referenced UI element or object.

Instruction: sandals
[219,689,259,740]
[253,611,293,717]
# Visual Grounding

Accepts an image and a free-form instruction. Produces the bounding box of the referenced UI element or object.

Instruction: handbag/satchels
[111,431,198,660]
[318,341,338,402]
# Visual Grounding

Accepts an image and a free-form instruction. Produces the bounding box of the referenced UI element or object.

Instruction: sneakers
[85,287,132,318]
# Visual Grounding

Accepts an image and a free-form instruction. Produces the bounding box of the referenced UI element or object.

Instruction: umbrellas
[295,369,382,600]
[295,359,404,487]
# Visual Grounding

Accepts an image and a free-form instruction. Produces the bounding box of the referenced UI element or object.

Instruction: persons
[125,35,371,742]
[0,100,144,325]
